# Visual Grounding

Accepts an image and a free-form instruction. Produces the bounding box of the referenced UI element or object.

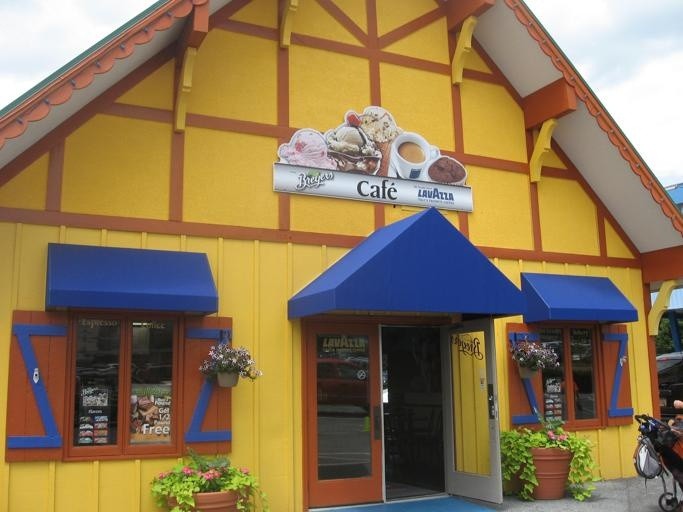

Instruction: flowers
[196,344,264,381]
[150,446,270,512]
[499,418,606,504]
[510,341,563,370]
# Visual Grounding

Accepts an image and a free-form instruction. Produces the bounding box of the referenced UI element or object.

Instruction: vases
[516,362,541,380]
[215,371,240,387]
[165,485,253,510]
[519,445,574,501]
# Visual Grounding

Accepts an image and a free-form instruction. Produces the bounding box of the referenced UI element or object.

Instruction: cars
[655,351,683,418]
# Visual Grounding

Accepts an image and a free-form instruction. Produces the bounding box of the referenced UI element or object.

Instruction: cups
[392,131,440,180]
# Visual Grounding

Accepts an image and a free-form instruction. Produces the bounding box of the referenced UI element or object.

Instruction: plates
[280,148,382,176]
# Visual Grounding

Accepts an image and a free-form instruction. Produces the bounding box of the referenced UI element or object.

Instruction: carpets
[311,496,495,511]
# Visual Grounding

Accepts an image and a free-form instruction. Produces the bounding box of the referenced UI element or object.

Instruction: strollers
[633,411,683,512]
